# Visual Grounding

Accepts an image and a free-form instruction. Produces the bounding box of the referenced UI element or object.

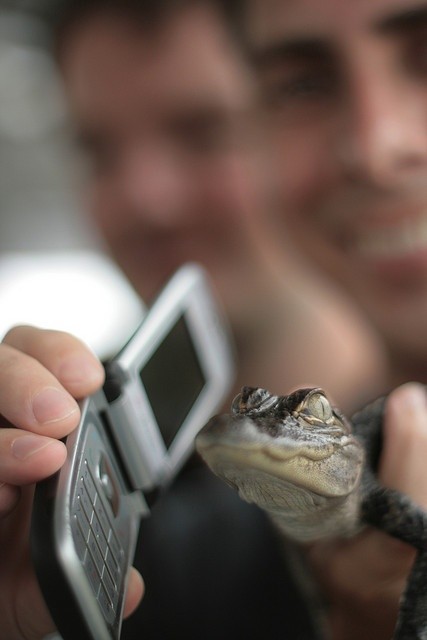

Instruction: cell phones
[28,259,236,640]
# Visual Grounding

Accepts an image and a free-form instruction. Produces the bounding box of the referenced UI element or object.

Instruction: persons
[37,1,389,638]
[0,0,424,639]
